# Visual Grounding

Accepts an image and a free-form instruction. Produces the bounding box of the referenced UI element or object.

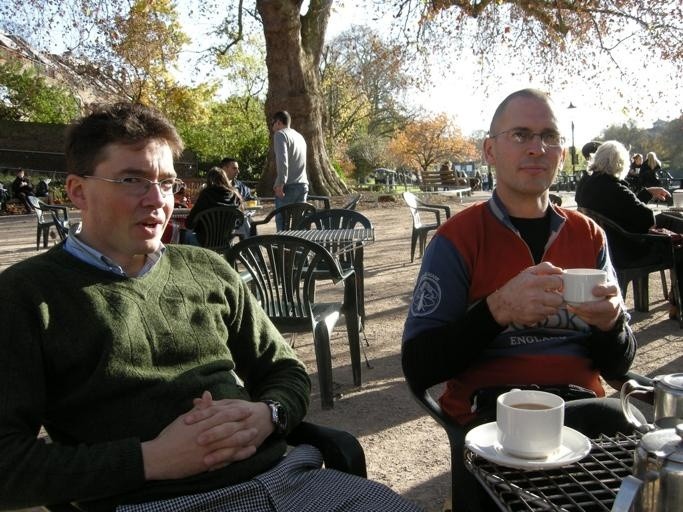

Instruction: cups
[494,388,564,460]
[246,199,257,206]
[551,268,608,308]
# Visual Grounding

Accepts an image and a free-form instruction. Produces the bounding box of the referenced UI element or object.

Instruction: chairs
[403,190,682,512]
[25,192,71,251]
[556,174,570,193]
[566,175,579,192]
[182,191,375,411]
[653,177,681,207]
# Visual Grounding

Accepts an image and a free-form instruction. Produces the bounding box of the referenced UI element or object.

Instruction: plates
[463,421,594,470]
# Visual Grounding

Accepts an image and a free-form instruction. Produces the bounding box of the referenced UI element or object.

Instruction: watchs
[263,398,289,432]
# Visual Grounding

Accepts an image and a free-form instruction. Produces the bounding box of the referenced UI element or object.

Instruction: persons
[268,110,310,229]
[401,92,643,438]
[441,159,452,191]
[12,169,52,213]
[578,140,683,320]
[220,156,256,261]
[1,104,426,511]
[186,166,241,248]
[174,182,191,207]
[576,142,601,208]
[628,153,643,184]
[639,150,661,203]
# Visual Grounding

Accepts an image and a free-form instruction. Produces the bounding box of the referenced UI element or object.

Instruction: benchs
[419,166,472,199]
[0,179,50,212]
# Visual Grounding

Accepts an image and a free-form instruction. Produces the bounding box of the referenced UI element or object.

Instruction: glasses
[489,126,567,149]
[83,175,186,197]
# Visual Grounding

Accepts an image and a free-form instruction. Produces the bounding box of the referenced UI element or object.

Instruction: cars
[373,169,419,185]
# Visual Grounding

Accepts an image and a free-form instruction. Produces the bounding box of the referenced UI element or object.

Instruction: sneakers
[668,302,680,320]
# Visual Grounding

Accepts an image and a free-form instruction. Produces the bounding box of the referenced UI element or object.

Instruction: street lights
[485,131,494,191]
[567,103,578,178]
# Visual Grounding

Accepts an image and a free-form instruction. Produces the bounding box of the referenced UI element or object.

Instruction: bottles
[627,424,683,512]
[649,372,683,435]
[671,188,683,211]
